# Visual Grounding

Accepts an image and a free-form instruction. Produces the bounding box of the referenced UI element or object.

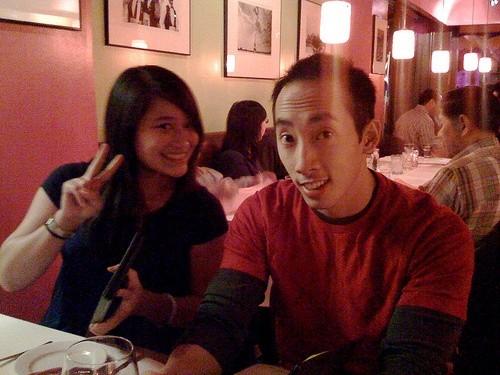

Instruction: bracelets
[156,293,176,328]
[44,218,71,240]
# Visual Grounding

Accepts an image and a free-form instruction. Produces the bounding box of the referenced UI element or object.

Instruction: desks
[0,314,165,375]
[368,154,452,189]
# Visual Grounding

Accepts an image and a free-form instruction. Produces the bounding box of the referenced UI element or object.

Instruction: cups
[365,147,380,171]
[62,334,139,375]
[390,143,432,175]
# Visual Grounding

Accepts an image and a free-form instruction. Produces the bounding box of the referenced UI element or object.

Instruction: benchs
[196,131,230,167]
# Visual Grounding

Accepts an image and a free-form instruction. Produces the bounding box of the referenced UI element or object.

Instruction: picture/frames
[224,0,281,81]
[371,14,388,75]
[105,0,192,57]
[298,0,334,67]
[0,0,82,31]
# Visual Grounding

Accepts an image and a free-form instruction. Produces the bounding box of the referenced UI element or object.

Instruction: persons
[215,101,268,181]
[394,88,443,148]
[146,54,475,375]
[422,85,500,241]
[0,65,230,354]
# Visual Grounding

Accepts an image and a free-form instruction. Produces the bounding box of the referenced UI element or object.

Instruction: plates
[15,340,108,375]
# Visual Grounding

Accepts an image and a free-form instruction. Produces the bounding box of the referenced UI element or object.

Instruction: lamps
[393,0,415,59]
[320,0,351,44]
[430,1,449,74]
[463,0,478,71]
[477,0,492,74]
